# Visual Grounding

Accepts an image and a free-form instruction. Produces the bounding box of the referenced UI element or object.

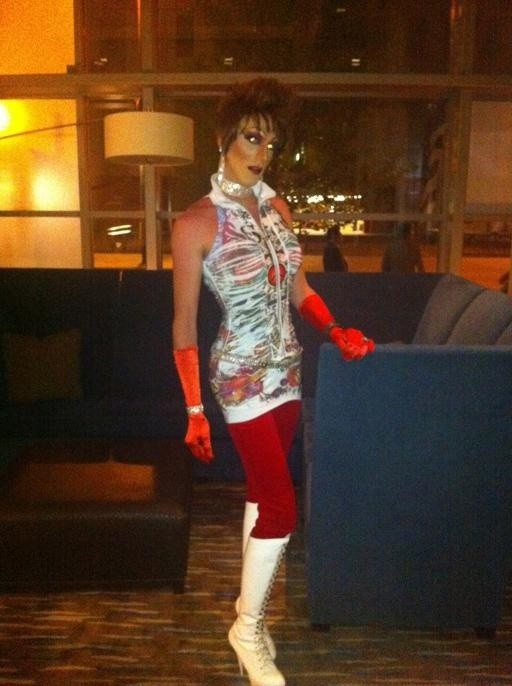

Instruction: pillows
[3,330,84,405]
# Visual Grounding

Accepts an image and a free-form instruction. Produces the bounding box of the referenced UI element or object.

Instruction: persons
[321,223,349,273]
[170,77,377,686]
[381,222,426,273]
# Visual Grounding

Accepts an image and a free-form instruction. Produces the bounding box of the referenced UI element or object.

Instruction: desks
[0,439,192,593]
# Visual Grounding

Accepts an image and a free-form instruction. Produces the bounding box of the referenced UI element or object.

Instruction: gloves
[298,292,375,362]
[171,346,215,465]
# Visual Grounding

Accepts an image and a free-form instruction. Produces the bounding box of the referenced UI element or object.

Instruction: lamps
[103,112,192,270]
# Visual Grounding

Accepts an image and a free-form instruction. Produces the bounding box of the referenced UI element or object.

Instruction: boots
[233,501,278,661]
[227,530,287,686]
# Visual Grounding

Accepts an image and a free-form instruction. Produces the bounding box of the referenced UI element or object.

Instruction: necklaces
[217,177,256,199]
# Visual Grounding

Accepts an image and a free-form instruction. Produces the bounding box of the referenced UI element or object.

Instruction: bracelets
[325,322,341,338]
[186,403,206,419]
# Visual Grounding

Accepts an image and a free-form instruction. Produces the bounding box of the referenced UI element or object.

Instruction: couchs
[0,269,512,636]
